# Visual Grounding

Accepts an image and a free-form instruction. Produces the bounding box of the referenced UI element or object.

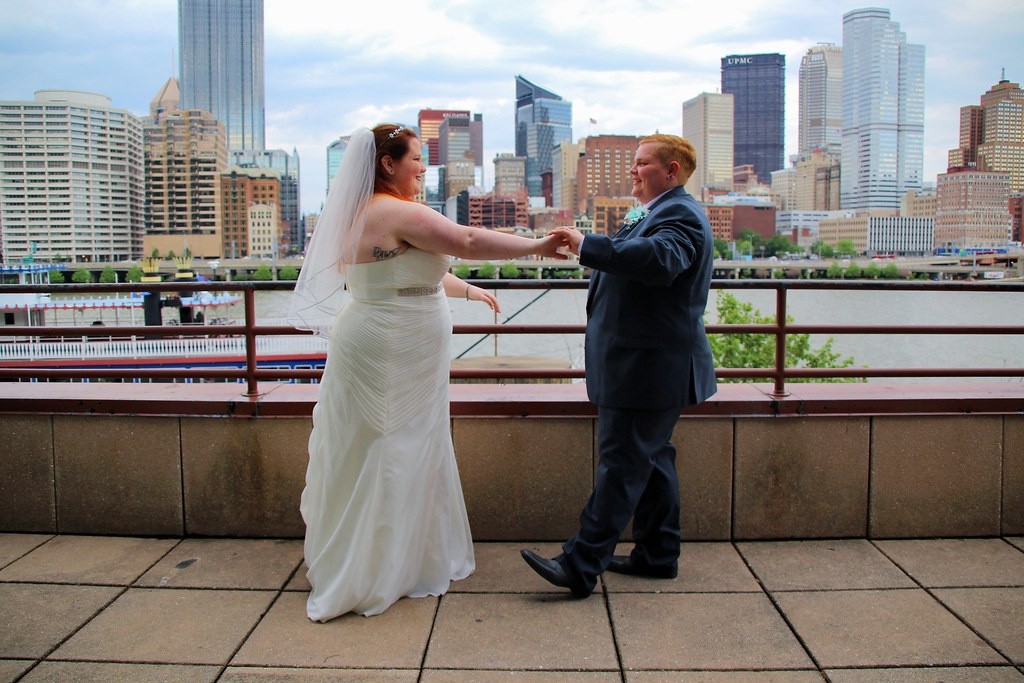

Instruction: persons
[520,134,717,598]
[290,125,569,623]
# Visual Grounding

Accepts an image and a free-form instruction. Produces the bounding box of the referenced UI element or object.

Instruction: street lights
[747,234,753,254]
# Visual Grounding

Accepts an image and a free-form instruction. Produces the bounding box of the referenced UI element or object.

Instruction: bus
[871,255,896,259]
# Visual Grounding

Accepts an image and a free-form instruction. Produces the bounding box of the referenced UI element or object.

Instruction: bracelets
[466,284,471,301]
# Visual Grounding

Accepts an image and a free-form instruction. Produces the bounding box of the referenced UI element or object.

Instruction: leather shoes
[606,555,678,579]
[520,549,592,598]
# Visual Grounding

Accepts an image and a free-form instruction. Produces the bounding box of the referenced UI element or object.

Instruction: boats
[0,264,329,384]
